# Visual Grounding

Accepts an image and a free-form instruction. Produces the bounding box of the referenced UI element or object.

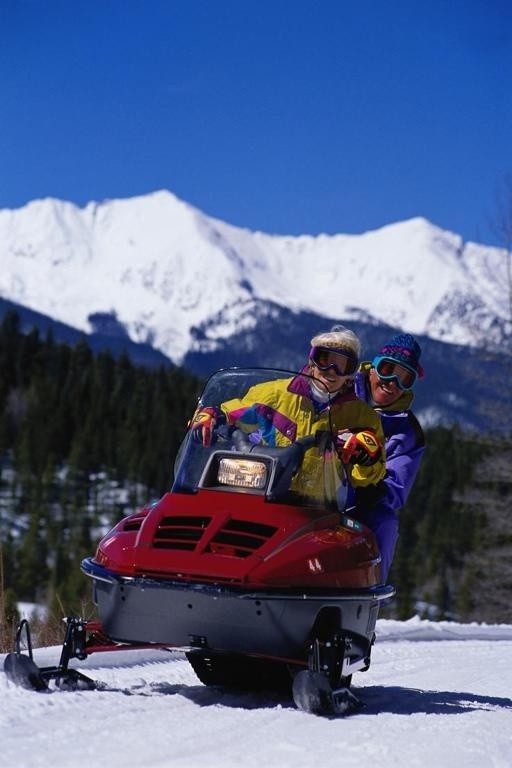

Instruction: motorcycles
[5,365,398,718]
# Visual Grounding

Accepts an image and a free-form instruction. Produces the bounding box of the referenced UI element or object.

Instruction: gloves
[342,431,382,466]
[189,405,227,448]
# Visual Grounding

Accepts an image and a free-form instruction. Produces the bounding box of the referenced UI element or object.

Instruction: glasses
[372,355,417,390]
[310,345,356,375]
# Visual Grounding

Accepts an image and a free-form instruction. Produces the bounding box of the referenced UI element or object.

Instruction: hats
[372,332,425,378]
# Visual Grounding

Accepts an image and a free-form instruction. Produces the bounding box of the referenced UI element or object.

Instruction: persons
[232,332,427,589]
[186,327,388,508]
